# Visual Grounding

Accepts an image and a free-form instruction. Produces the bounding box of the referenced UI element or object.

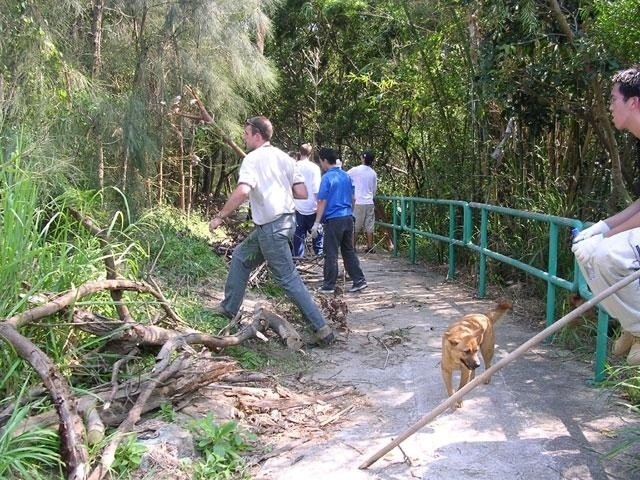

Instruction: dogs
[441,299,513,412]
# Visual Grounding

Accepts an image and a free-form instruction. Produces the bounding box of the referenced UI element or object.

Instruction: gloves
[570,234,605,265]
[310,223,320,238]
[574,221,612,242]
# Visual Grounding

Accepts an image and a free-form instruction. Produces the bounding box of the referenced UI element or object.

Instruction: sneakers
[612,328,635,356]
[316,287,335,295]
[349,280,368,292]
[306,326,334,346]
[205,304,237,319]
[626,336,640,366]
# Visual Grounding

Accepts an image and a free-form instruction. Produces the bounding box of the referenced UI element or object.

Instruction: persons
[204,114,335,350]
[570,64,639,368]
[287,143,380,295]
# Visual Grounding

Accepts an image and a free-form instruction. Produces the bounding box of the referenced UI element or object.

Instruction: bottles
[570,227,596,282]
